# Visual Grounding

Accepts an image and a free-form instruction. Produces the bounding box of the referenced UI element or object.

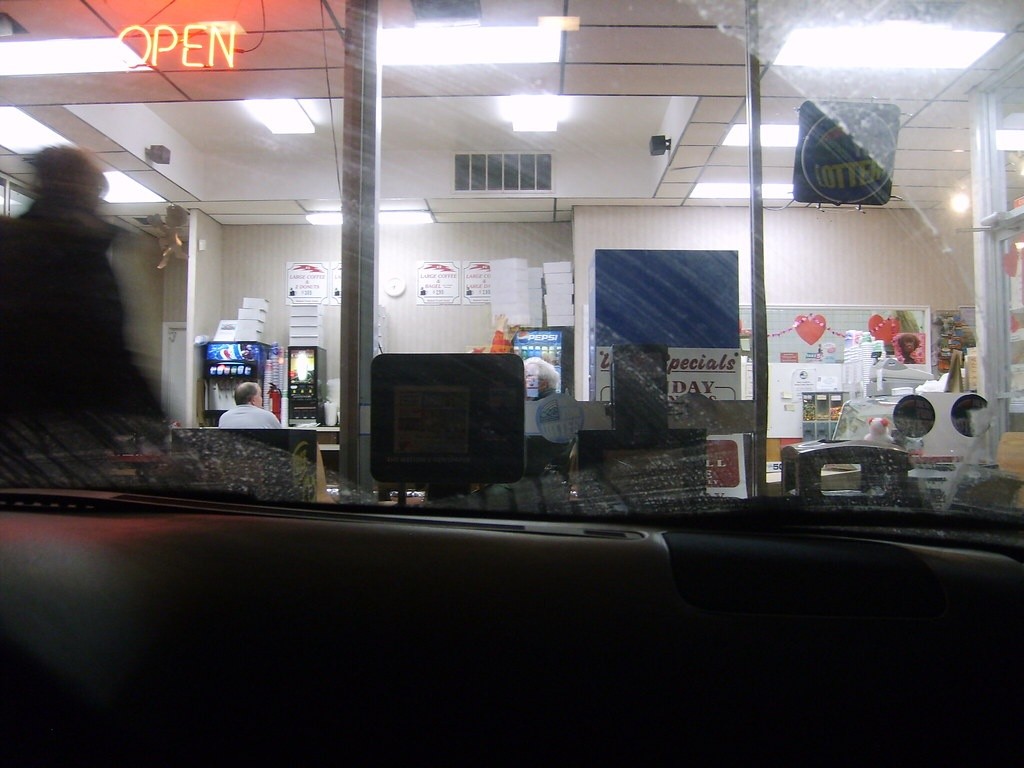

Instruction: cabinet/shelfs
[289,304,324,347]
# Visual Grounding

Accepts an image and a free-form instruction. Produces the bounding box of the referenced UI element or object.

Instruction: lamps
[144,145,170,164]
[650,135,671,155]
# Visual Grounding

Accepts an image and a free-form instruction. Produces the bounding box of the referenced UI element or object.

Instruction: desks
[171,427,340,501]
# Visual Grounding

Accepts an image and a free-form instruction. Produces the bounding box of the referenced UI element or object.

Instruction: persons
[0,144,168,425]
[219,382,281,428]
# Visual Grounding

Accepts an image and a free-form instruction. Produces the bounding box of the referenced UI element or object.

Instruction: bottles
[513,346,561,361]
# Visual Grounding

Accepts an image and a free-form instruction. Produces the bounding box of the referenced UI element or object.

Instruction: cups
[323,401,338,426]
[262,342,288,428]
[843,330,886,396]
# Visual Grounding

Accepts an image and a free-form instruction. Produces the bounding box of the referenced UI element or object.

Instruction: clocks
[384,276,405,297]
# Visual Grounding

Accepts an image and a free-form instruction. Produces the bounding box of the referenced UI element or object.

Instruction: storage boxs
[490,258,574,329]
[236,298,268,341]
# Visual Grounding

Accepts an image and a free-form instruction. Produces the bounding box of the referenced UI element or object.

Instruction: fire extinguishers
[268,383,282,425]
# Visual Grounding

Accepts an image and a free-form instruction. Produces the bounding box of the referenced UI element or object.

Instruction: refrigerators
[512,327,575,400]
